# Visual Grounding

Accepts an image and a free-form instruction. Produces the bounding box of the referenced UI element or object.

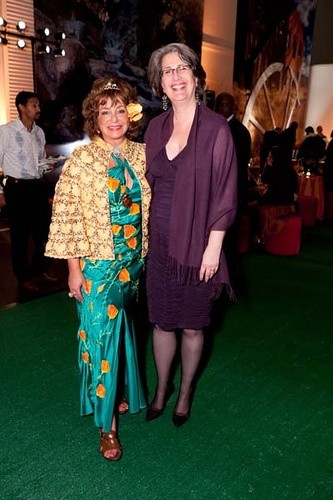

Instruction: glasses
[159,64,193,77]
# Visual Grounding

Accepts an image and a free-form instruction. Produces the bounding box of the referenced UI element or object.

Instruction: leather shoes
[21,279,38,291]
[43,267,61,281]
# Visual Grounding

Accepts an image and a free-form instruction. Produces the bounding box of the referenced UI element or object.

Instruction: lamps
[0,16,66,57]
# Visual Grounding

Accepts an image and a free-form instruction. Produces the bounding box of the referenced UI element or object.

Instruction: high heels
[145,387,169,420]
[173,390,193,426]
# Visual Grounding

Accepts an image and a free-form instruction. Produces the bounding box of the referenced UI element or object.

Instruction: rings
[68,292,76,298]
[211,269,215,271]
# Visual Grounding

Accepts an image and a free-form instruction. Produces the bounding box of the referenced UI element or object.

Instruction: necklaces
[122,172,132,208]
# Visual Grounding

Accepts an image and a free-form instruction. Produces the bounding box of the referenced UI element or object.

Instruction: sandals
[115,398,128,414]
[100,428,121,461]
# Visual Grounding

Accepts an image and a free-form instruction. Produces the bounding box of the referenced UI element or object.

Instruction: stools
[259,203,302,256]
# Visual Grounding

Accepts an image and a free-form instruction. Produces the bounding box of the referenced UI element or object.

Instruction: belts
[8,176,36,183]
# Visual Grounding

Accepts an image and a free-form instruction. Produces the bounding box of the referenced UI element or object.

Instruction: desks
[297,173,325,226]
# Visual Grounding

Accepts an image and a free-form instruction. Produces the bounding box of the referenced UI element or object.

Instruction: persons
[215,91,251,267]
[259,121,333,218]
[145,43,239,428]
[0,90,63,293]
[43,77,152,459]
